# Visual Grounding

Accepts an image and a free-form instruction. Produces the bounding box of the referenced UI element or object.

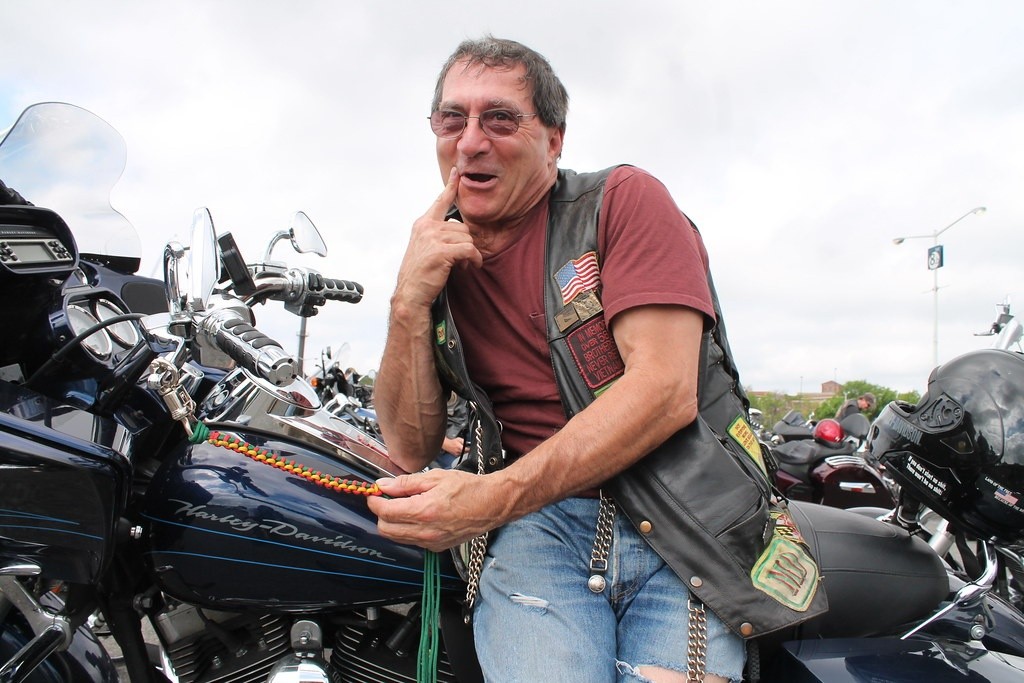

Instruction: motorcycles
[1,101,1024,683]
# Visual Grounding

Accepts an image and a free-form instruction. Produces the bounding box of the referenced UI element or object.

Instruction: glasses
[428,109,538,139]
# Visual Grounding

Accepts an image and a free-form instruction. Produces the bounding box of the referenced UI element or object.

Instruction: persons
[834,392,876,424]
[363,35,831,681]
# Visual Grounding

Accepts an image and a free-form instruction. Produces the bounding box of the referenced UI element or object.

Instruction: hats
[863,392,876,410]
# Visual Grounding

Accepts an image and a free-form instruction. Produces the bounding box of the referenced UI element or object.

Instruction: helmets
[866,348,1024,545]
[813,419,843,449]
